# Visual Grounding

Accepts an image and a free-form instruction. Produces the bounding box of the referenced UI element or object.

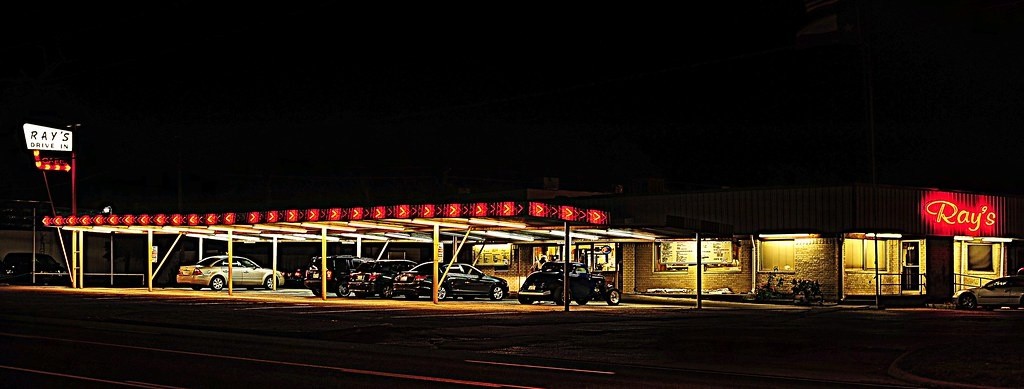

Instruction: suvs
[347,260,418,299]
[304,255,375,297]
[0,252,69,282]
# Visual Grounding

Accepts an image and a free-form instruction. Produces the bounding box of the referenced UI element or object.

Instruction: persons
[580,256,584,263]
[538,256,548,271]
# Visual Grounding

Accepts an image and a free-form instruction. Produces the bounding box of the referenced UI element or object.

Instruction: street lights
[104,206,114,288]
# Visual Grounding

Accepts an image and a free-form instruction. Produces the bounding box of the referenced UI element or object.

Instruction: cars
[517,262,621,306]
[392,262,509,301]
[952,276,1024,310]
[176,255,285,292]
[287,267,305,288]
